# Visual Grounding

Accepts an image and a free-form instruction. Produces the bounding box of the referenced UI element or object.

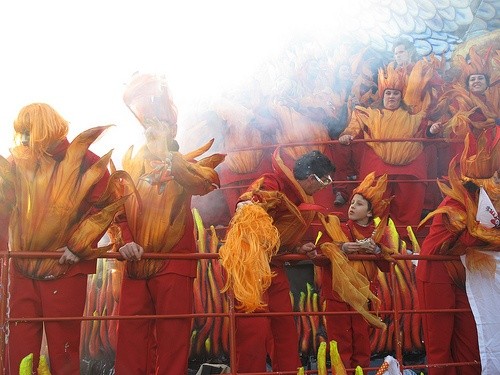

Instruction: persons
[205,42,500,375]
[108,99,226,375]
[0,102,118,375]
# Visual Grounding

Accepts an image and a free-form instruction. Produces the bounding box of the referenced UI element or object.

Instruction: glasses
[314,173,332,185]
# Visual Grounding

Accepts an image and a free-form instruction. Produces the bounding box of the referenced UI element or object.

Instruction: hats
[459,44,493,84]
[377,62,406,97]
[351,171,395,217]
[133,74,177,125]
[459,126,500,178]
